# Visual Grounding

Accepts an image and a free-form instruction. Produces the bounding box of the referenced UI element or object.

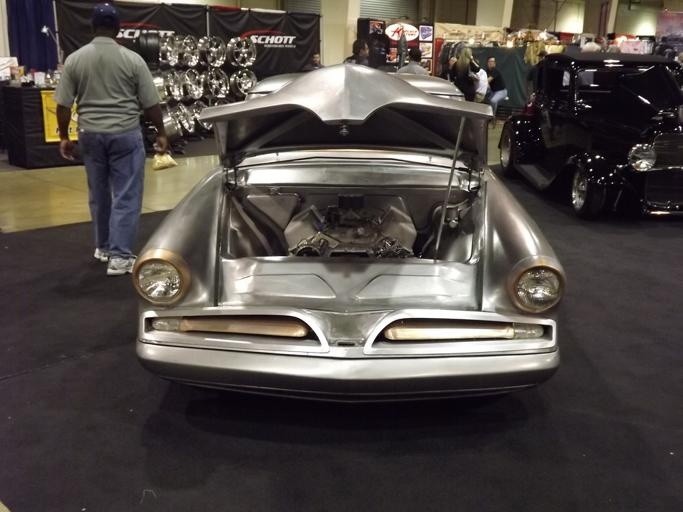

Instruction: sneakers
[94,248,108,262]
[107,256,138,276]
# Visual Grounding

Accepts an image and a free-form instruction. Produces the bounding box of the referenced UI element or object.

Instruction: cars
[128,61,568,407]
[496,51,682,223]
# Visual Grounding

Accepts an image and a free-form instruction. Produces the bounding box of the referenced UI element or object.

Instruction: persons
[343,40,370,68]
[302,51,326,72]
[651,37,672,57]
[604,44,622,54]
[580,42,603,55]
[468,58,489,105]
[452,49,481,102]
[439,56,461,92]
[486,56,507,130]
[396,48,431,78]
[56,4,170,278]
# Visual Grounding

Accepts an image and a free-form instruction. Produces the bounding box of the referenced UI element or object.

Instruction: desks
[0,80,84,170]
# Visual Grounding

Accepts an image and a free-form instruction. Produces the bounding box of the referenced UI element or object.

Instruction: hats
[92,3,118,24]
